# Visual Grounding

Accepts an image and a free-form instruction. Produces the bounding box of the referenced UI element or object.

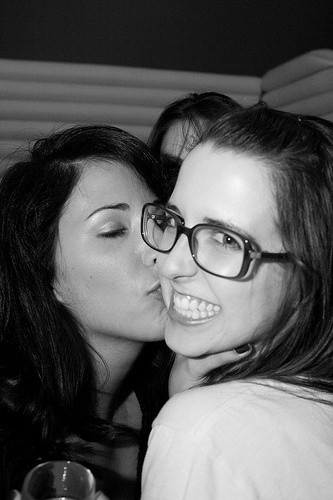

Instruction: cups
[23,462,95,500]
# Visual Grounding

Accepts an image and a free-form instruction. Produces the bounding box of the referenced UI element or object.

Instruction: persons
[146,91,246,161]
[0,125,252,500]
[141,100,333,500]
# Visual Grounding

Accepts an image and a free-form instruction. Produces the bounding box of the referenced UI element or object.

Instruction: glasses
[140,203,293,280]
